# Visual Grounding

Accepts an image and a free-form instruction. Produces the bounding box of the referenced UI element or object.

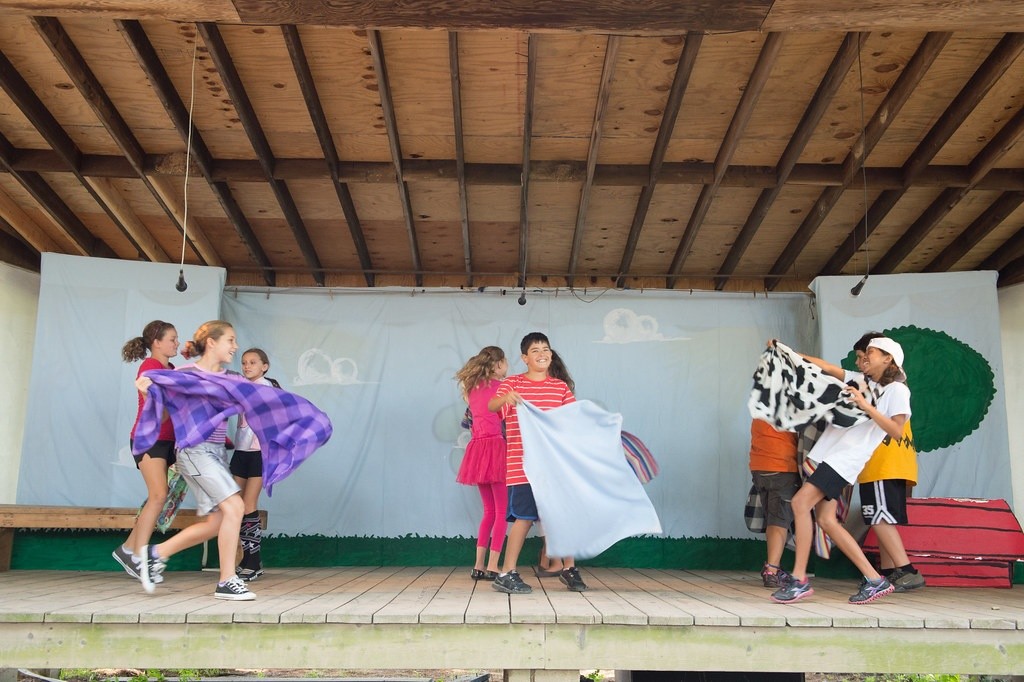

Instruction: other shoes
[535,567,564,577]
[538,565,546,572]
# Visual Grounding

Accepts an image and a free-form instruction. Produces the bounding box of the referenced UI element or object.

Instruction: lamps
[849,32,869,296]
[176,31,196,292]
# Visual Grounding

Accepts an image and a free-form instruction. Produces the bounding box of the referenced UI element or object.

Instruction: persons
[112,320,180,583]
[535,350,574,577]
[853,333,925,591]
[134,320,255,600]
[748,419,802,587]
[766,337,912,604]
[487,333,587,593]
[454,346,508,579]
[230,348,282,581]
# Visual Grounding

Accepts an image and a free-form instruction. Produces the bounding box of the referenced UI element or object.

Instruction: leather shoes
[485,570,498,580]
[470,569,485,580]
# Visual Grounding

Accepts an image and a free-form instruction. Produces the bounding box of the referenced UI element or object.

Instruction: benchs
[0,504,269,574]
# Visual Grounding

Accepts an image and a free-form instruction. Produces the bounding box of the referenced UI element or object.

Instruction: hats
[866,337,907,382]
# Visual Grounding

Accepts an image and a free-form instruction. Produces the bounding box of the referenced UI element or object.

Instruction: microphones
[176,275,187,292]
[851,275,868,296]
[518,291,526,305]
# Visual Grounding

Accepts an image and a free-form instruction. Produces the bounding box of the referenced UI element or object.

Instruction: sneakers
[492,569,531,594]
[770,574,814,604]
[140,543,167,594]
[886,568,925,591]
[215,576,256,600]
[236,566,242,574]
[559,567,588,591]
[848,575,895,604]
[238,566,264,580]
[111,544,163,583]
[761,563,791,587]
